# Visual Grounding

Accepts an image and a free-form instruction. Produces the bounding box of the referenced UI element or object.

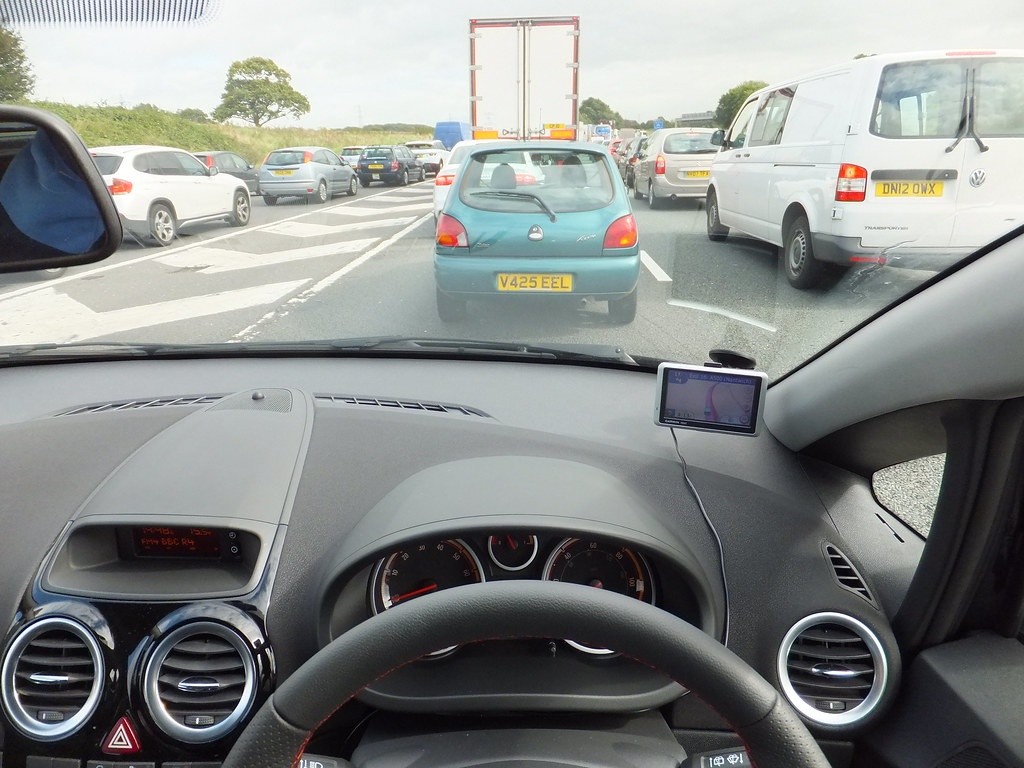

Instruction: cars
[588,128,647,186]
[434,140,544,224]
[631,128,730,208]
[434,146,641,324]
[416,149,451,178]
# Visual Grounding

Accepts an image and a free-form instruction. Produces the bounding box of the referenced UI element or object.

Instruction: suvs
[341,145,426,187]
[258,147,358,205]
[87,145,251,247]
[405,140,447,154]
[194,152,261,197]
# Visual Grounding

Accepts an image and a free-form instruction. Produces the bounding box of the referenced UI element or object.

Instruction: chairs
[490,163,516,189]
[560,156,586,188]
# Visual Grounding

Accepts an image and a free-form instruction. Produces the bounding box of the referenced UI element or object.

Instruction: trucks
[468,15,580,147]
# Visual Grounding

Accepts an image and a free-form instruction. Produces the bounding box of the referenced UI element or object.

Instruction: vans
[706,50,1023,290]
